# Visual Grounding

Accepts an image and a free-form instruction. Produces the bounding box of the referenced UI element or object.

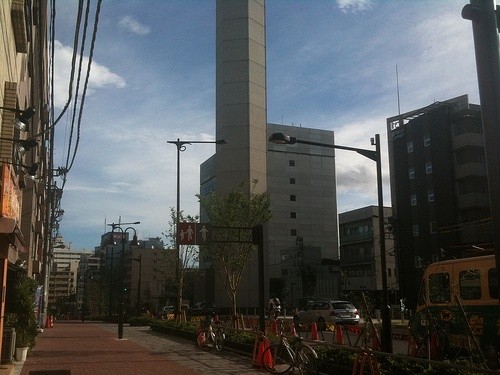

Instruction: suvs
[293,300,360,331]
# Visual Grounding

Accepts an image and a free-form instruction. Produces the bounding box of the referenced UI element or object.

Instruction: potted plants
[0,266,38,364]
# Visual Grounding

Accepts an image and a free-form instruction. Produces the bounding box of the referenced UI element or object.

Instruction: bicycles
[196,321,225,352]
[262,324,319,375]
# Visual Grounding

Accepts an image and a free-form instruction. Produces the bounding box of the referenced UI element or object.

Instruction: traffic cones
[271,318,280,335]
[311,322,319,341]
[337,325,345,345]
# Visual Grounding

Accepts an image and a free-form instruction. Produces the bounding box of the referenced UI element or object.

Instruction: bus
[418,254,500,354]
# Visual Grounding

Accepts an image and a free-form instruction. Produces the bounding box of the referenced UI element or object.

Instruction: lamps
[0,160,40,181]
[0,136,40,153]
[0,104,37,132]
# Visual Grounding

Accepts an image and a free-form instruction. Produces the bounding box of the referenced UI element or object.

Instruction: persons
[268,295,281,319]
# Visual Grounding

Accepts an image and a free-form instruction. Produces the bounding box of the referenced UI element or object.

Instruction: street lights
[268,131,391,353]
[167,138,228,322]
[107,227,141,339]
